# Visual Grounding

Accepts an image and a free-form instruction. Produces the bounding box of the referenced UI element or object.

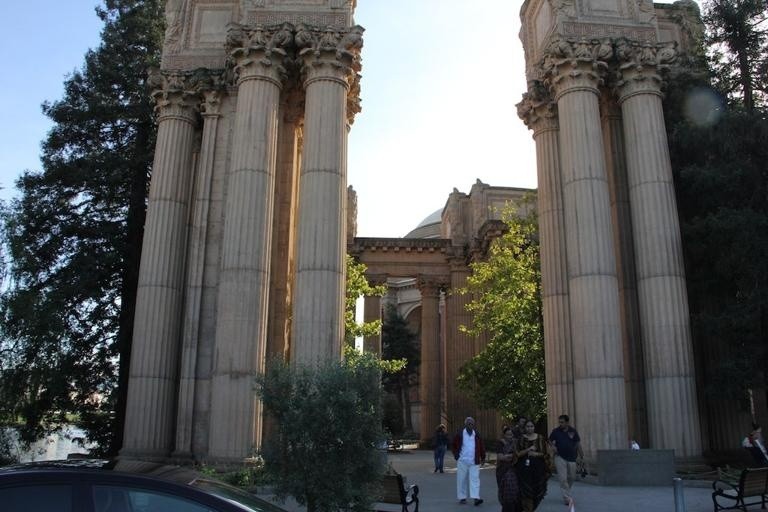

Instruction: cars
[0,459,288,511]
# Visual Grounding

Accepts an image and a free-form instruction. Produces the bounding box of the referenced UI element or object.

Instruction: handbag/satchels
[576,455,585,475]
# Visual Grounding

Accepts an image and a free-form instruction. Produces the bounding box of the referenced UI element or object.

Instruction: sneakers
[461,499,483,506]
[434,468,444,473]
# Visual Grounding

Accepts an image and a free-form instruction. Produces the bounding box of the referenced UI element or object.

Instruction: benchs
[387,440,403,454]
[373,473,419,512]
[712,467,768,512]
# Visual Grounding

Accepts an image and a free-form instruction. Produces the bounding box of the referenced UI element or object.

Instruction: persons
[496,417,552,512]
[433,424,448,474]
[631,439,640,449]
[742,423,768,468]
[453,417,486,506]
[548,415,584,506]
